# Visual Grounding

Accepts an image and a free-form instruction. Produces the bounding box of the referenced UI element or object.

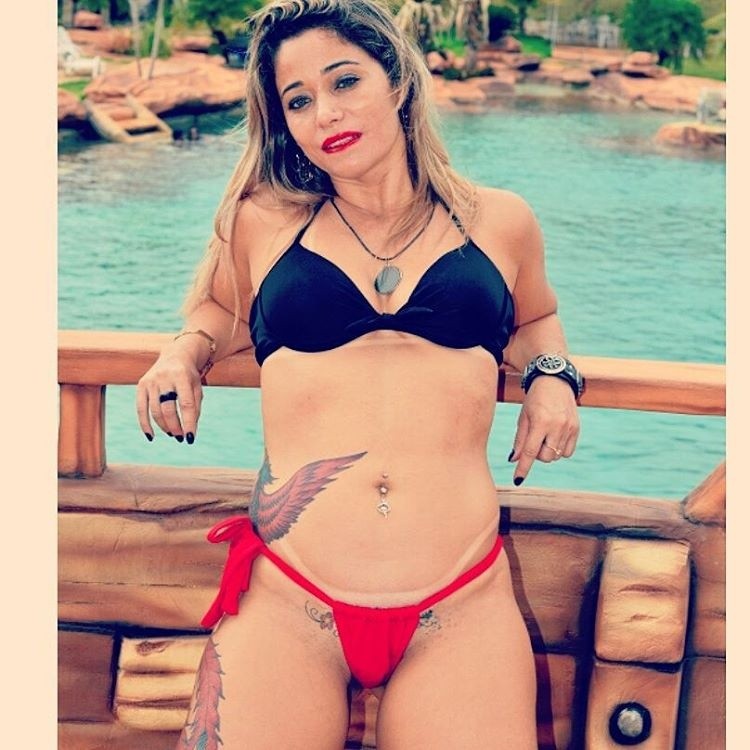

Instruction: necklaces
[329,197,435,295]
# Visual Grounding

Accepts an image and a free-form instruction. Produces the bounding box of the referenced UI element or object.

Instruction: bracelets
[174,330,217,374]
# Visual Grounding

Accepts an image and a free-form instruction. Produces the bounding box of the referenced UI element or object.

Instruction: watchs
[520,353,584,401]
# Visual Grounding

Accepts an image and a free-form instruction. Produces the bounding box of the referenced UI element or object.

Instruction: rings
[546,443,562,457]
[159,391,177,403]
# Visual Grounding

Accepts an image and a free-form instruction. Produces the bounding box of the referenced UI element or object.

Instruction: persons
[134,0,581,750]
